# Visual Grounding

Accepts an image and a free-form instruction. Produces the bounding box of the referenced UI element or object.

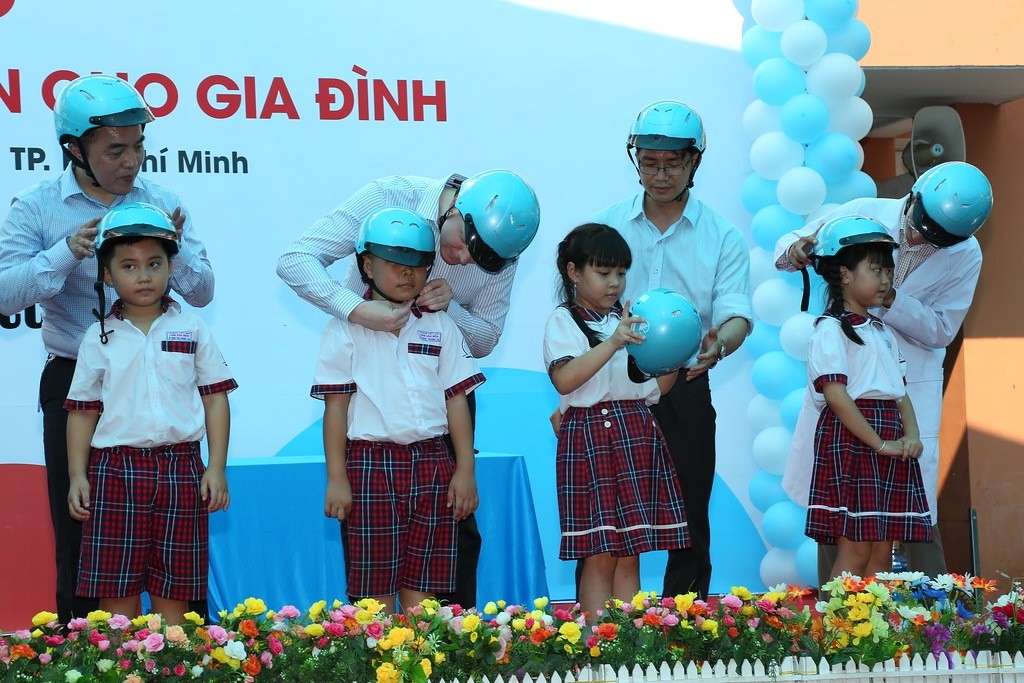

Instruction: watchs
[719,339,726,360]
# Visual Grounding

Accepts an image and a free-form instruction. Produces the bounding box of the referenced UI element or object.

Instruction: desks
[142,454,555,625]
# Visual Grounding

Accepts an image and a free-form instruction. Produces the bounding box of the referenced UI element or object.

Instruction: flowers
[0,570,1024,683]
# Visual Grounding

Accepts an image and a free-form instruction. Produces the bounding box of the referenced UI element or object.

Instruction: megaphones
[902,106,966,183]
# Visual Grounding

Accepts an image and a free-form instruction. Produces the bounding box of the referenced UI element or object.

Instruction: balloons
[734,0,877,595]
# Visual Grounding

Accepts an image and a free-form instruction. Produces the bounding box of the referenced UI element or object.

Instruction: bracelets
[874,440,885,452]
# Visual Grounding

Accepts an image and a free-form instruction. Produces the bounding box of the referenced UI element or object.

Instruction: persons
[775,159,992,599]
[576,99,753,603]
[800,217,934,600]
[543,224,692,625]
[65,201,238,630]
[309,205,478,619]
[277,170,540,614]
[0,74,215,638]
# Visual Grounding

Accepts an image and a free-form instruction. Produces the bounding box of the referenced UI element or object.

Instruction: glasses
[907,200,938,249]
[639,156,692,176]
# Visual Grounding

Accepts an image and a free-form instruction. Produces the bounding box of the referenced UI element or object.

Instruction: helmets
[625,101,706,154]
[811,215,899,275]
[92,202,181,261]
[625,287,703,384]
[910,161,993,247]
[353,206,436,283]
[54,74,155,171]
[453,169,540,276]
